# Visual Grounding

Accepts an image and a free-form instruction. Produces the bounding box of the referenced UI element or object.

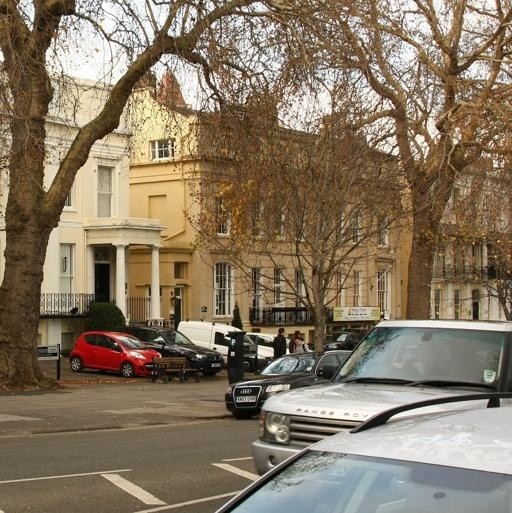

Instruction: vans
[175,319,258,369]
[344,328,373,337]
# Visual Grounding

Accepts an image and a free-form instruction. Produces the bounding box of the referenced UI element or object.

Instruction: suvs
[252,319,512,474]
[215,392,511,513]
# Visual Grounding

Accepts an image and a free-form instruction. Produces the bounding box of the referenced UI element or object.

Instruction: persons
[289,330,308,353]
[272,328,287,358]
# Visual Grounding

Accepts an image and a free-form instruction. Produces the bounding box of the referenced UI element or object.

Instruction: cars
[224,349,355,419]
[116,324,225,376]
[307,332,362,350]
[70,332,163,377]
[245,332,313,361]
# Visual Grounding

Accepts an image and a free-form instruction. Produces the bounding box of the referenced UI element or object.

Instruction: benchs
[145,355,204,383]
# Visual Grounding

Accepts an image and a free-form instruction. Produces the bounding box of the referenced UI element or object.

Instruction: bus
[248,305,334,325]
